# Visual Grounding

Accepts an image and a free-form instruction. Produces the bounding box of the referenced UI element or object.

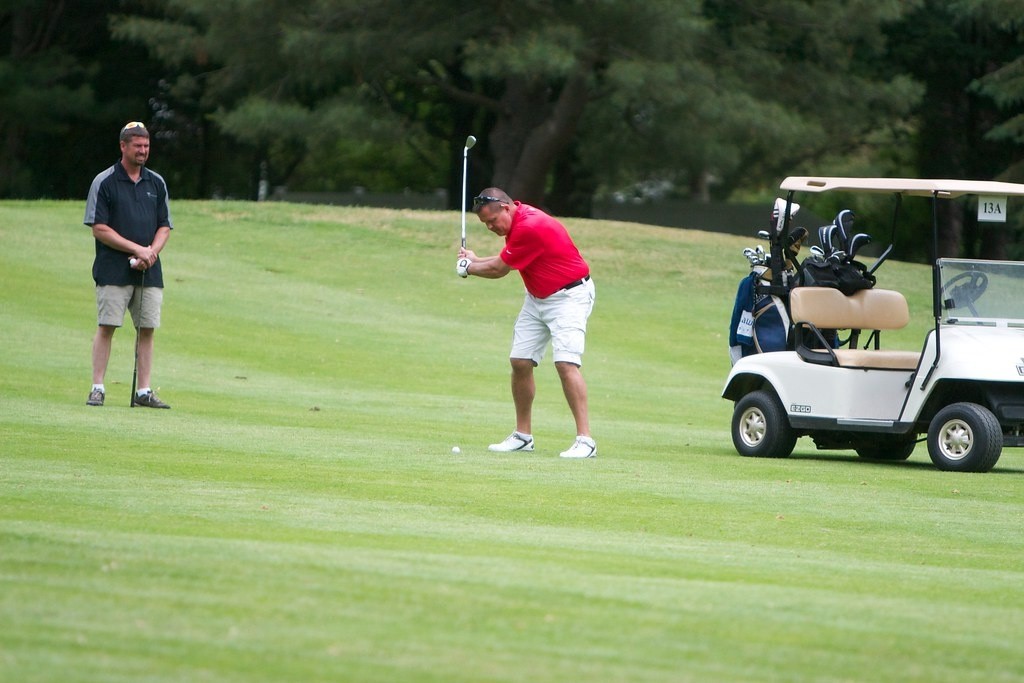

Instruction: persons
[84,122,174,409]
[457,188,597,457]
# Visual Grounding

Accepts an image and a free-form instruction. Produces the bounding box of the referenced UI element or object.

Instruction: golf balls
[452,447,460,453]
[129,258,137,264]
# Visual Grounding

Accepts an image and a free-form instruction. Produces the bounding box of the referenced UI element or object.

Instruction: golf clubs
[462,136,476,278]
[130,267,146,407]
[743,245,771,266]
[757,230,774,247]
[809,245,825,256]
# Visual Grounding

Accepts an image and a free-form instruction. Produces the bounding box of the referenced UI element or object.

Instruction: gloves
[455,257,471,277]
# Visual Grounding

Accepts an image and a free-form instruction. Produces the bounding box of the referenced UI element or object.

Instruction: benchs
[789,287,922,370]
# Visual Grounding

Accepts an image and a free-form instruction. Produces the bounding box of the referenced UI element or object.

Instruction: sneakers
[487,430,535,453]
[85,387,106,406]
[558,434,598,458]
[134,387,171,409]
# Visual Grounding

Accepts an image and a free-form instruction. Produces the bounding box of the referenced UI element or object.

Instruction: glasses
[121,122,146,136]
[473,196,510,208]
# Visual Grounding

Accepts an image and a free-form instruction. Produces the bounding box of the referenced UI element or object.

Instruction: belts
[554,274,590,292]
